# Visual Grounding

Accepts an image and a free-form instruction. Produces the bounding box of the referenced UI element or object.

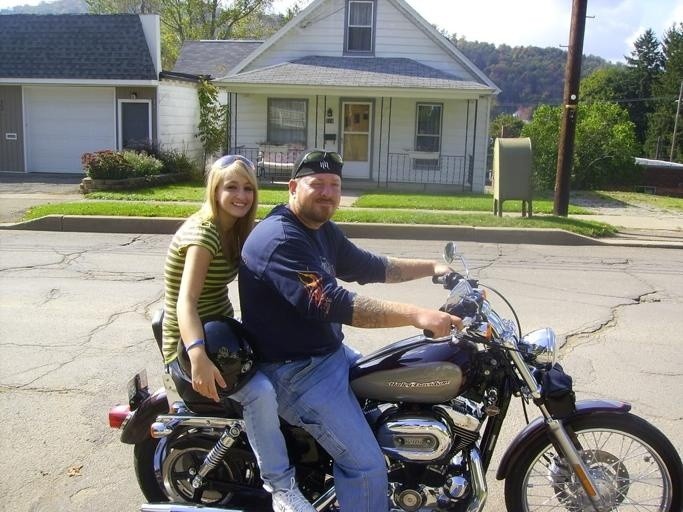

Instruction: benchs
[257,143,305,181]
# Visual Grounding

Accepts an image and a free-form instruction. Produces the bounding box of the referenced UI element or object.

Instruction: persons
[231,146,466,510]
[158,151,322,511]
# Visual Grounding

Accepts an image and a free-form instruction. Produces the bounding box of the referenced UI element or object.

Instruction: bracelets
[185,338,205,352]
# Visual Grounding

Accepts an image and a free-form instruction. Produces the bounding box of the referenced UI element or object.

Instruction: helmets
[177,314,257,399]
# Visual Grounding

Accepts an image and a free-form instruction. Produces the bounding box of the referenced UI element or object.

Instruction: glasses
[219,155,255,169]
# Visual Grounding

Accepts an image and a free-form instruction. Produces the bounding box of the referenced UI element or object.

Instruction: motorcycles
[108,241,681,512]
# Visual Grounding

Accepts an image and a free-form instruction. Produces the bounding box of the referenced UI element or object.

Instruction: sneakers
[272,482,318,512]
